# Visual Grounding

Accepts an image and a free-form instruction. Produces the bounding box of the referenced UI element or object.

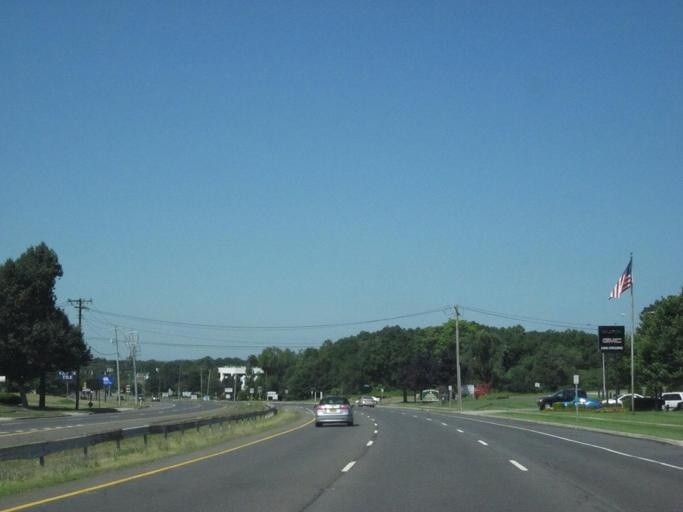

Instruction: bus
[81,389,96,400]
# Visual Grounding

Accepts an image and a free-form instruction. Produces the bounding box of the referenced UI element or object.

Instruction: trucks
[440,384,489,401]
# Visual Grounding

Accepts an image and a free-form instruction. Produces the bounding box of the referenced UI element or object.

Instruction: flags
[608,258,633,300]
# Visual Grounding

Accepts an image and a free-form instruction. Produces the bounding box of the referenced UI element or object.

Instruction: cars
[537,389,683,411]
[117,391,127,401]
[355,394,378,409]
[312,396,354,427]
[152,394,160,401]
[138,393,145,402]
